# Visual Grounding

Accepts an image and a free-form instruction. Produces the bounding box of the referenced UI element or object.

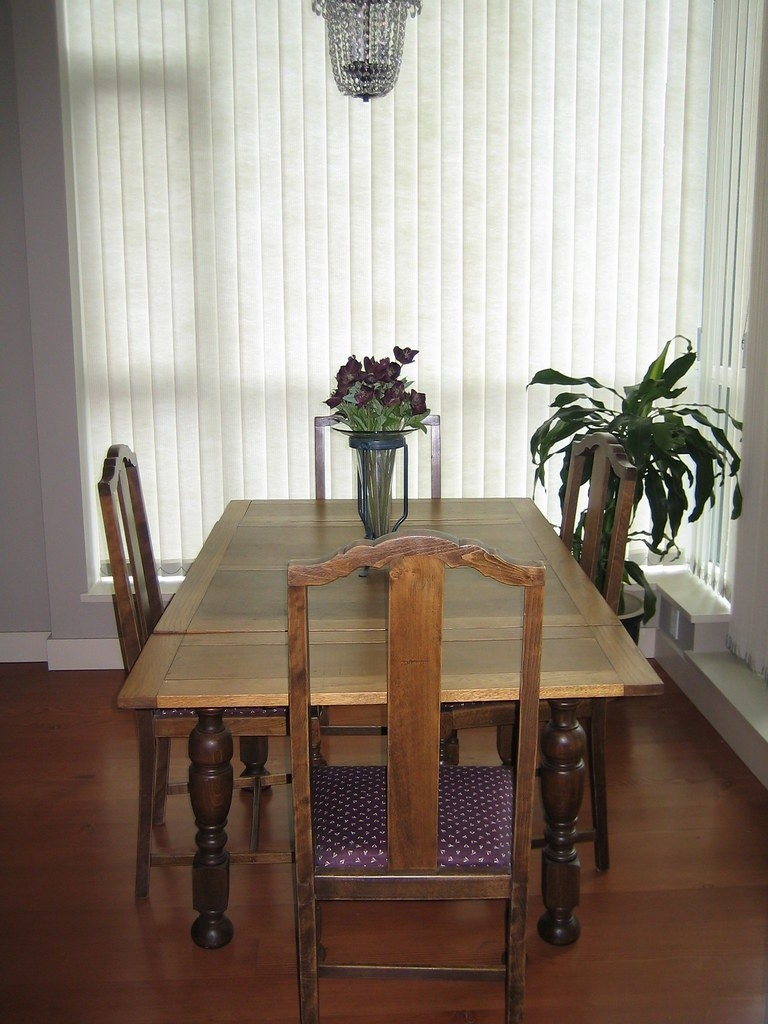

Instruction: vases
[348,422,409,578]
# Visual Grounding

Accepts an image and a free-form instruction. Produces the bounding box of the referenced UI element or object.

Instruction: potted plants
[525,335,744,647]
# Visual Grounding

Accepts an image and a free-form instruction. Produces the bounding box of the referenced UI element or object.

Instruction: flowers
[323,346,431,434]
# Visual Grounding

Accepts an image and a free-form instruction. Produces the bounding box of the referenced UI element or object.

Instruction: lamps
[312,0,423,102]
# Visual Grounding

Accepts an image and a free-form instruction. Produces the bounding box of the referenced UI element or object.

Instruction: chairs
[98,444,322,900]
[440,432,638,872]
[314,412,441,500]
[286,529,545,1024]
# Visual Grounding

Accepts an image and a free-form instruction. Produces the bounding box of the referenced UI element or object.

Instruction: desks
[116,497,665,948]
